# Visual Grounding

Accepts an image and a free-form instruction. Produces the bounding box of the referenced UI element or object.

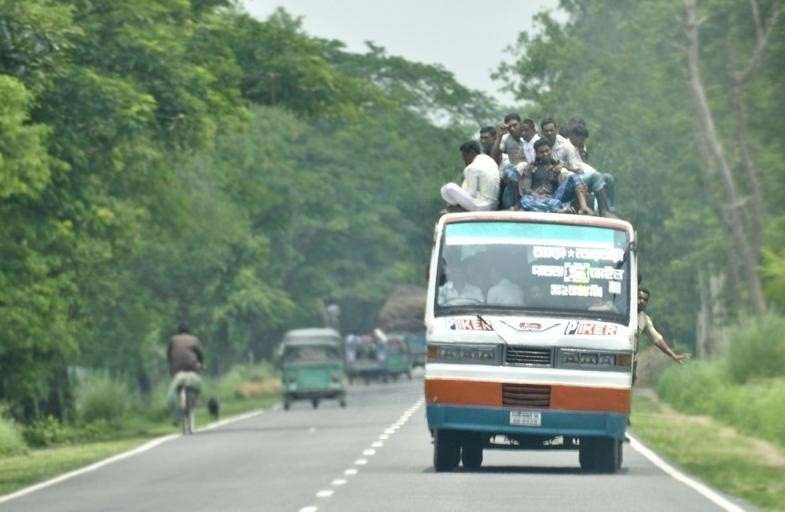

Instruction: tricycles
[273,324,427,411]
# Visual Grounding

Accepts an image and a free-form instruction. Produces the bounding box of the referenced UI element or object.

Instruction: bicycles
[171,366,204,440]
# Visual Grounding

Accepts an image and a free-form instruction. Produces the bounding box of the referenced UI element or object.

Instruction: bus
[414,207,644,476]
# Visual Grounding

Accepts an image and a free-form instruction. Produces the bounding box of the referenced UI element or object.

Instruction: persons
[345,324,407,370]
[635,285,694,367]
[322,296,340,332]
[433,113,625,319]
[165,322,209,416]
